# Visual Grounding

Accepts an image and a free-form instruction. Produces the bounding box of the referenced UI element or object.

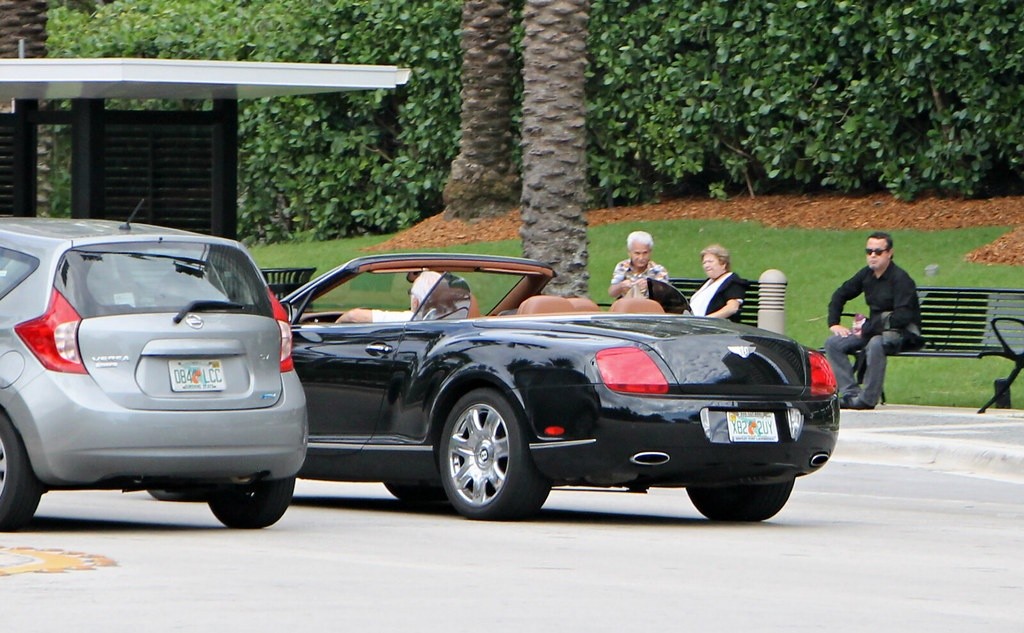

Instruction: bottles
[853,314,866,336]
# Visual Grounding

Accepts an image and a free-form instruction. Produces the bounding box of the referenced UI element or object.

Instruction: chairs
[423,288,666,318]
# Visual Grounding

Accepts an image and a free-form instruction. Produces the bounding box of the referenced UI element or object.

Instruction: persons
[608,230,668,302]
[824,231,924,410]
[689,245,745,323]
[333,271,449,324]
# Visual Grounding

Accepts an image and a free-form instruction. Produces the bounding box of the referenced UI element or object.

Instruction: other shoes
[839,397,874,409]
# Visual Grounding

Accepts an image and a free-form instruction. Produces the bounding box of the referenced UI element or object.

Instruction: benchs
[593,277,759,329]
[815,286,1024,413]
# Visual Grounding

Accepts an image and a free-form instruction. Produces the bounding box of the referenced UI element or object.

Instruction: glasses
[866,248,888,255]
[407,289,416,298]
